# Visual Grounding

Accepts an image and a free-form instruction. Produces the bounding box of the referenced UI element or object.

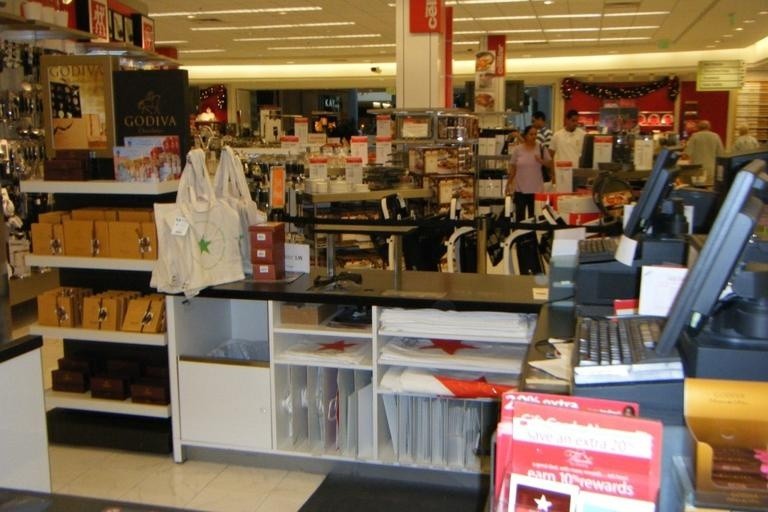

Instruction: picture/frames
[39,53,117,161]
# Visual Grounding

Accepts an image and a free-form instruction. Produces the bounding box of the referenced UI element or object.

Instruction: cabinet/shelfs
[250,180,540,276]
[22,181,182,463]
[171,291,539,481]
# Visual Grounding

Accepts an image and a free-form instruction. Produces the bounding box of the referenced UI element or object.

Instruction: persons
[505,125,554,222]
[730,124,759,154]
[512,111,553,149]
[680,119,726,189]
[550,108,587,185]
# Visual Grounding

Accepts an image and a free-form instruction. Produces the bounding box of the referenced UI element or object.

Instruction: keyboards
[578,239,617,261]
[573,312,638,385]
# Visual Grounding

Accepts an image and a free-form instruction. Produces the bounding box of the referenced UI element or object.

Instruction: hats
[734,123,750,130]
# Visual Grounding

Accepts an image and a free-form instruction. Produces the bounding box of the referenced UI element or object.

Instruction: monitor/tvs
[654,169,767,365]
[623,149,682,240]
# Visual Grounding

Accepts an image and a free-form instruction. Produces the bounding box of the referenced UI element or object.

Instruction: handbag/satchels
[148,145,269,299]
[503,184,513,220]
[540,143,553,183]
